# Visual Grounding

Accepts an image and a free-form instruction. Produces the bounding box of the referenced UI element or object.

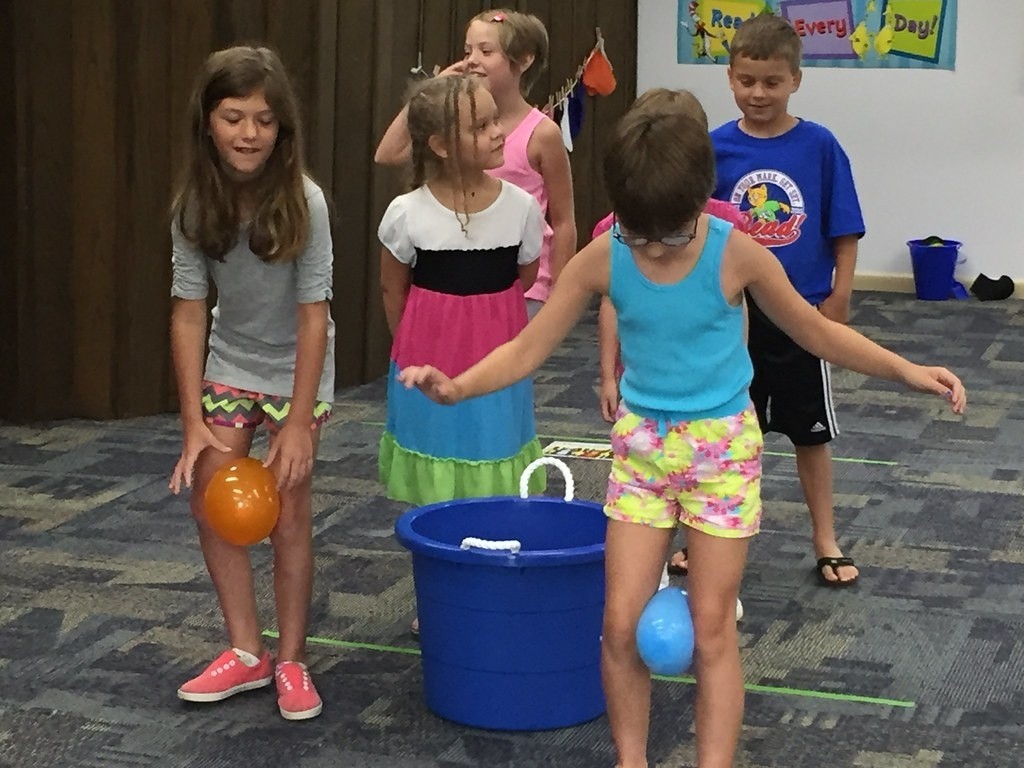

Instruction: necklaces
[470,191,476,197]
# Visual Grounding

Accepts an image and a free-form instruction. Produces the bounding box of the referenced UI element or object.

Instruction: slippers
[668,545,688,575]
[815,552,859,586]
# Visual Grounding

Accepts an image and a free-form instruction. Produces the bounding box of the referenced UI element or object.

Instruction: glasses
[612,212,698,247]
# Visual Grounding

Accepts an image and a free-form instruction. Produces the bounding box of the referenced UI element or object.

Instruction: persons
[373,6,578,327]
[374,73,549,641]
[396,107,967,767]
[673,11,866,590]
[163,47,338,723]
[591,87,756,623]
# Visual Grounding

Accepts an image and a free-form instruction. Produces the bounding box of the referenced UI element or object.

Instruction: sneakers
[176,647,324,720]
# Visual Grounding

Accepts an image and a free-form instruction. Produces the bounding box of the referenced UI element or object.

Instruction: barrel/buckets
[393,456,612,730]
[906,239,963,301]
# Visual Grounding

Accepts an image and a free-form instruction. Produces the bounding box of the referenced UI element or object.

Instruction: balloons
[633,586,696,677]
[203,459,280,549]
